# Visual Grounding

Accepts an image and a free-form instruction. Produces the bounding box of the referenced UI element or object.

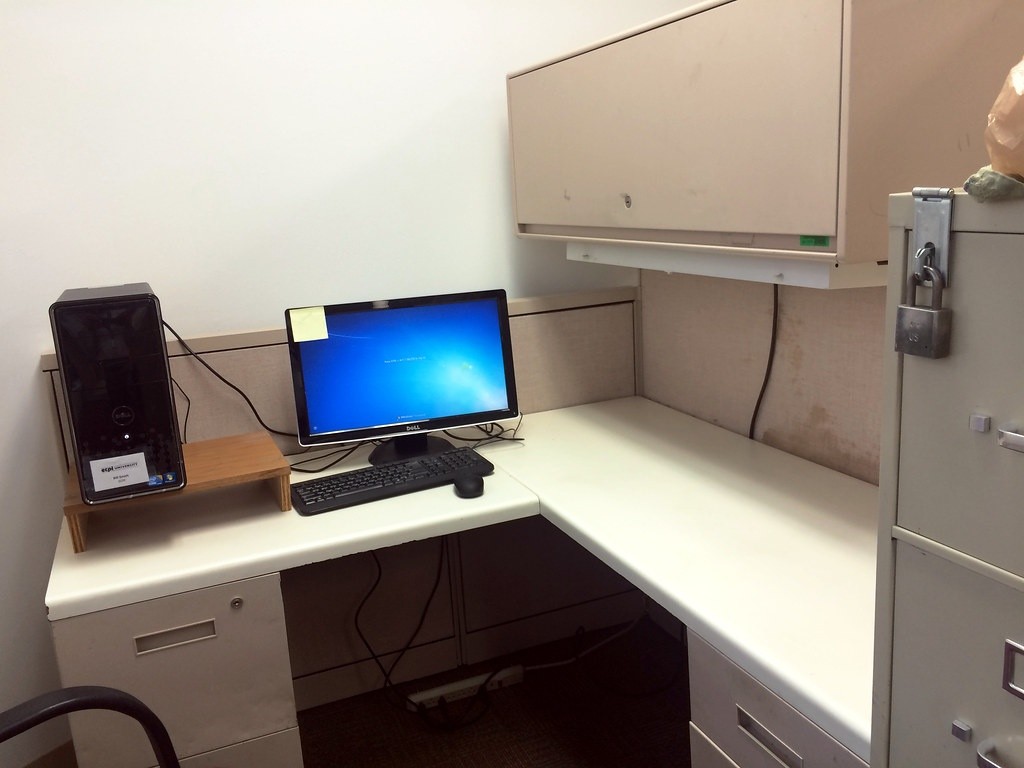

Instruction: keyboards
[289,446,495,517]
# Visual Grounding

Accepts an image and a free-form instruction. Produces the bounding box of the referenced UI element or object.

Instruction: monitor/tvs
[285,289,519,467]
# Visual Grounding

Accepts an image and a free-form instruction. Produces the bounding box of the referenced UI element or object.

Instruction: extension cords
[406,666,526,711]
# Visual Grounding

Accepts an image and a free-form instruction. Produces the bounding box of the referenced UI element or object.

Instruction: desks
[44,395,879,768]
[63,432,287,554]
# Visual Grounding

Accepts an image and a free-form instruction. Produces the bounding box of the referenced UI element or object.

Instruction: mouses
[452,472,484,499]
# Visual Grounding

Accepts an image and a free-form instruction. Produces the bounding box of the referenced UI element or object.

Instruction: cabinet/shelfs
[867,187,1024,768]
[506,0,1024,290]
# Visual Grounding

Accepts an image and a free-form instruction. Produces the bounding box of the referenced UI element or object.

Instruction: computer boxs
[49,282,187,507]
